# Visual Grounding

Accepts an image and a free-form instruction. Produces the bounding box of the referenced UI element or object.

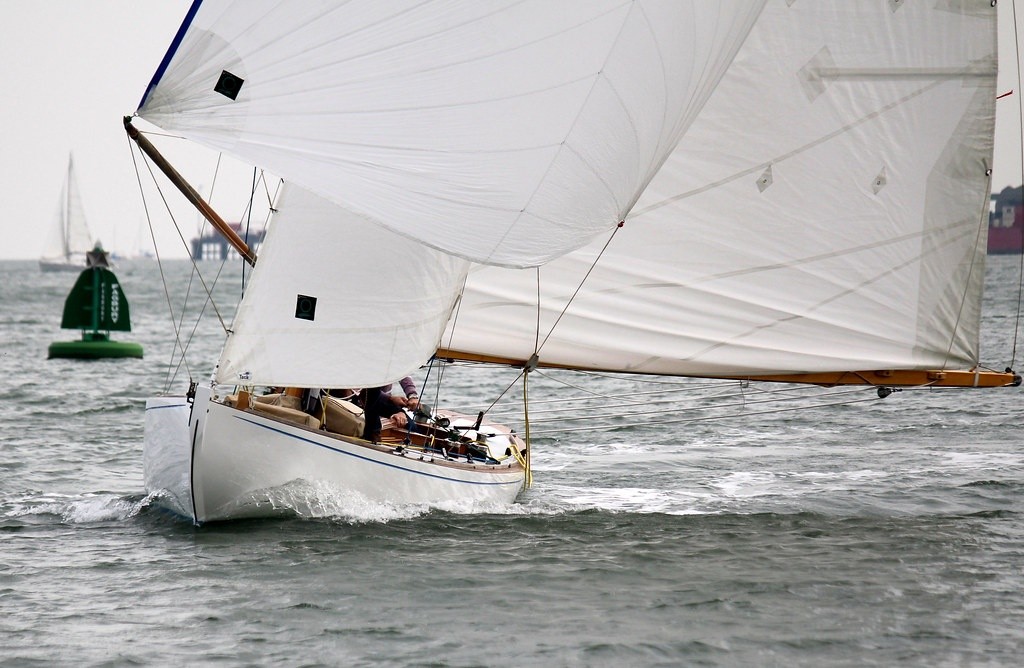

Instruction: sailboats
[38,151,118,273]
[122,0,1024,528]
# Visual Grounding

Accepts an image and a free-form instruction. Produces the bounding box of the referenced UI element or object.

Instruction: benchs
[312,396,398,438]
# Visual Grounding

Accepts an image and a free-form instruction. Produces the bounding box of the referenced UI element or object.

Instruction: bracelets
[409,396,418,399]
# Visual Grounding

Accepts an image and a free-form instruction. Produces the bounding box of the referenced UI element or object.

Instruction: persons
[381,376,421,412]
[301,388,408,445]
